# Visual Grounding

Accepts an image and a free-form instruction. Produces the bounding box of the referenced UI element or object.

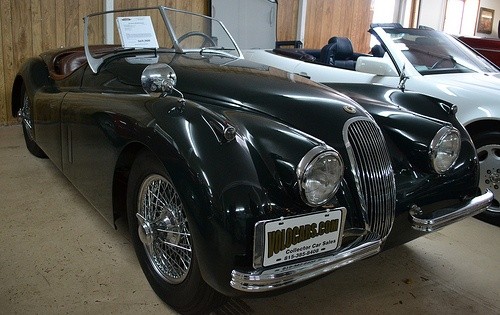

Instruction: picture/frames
[477,7,495,35]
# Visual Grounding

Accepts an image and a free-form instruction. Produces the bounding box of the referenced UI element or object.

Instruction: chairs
[320,36,357,71]
[61,47,121,77]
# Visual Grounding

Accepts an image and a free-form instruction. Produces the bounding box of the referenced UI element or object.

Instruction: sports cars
[10,5,493,315]
[210,20,500,228]
